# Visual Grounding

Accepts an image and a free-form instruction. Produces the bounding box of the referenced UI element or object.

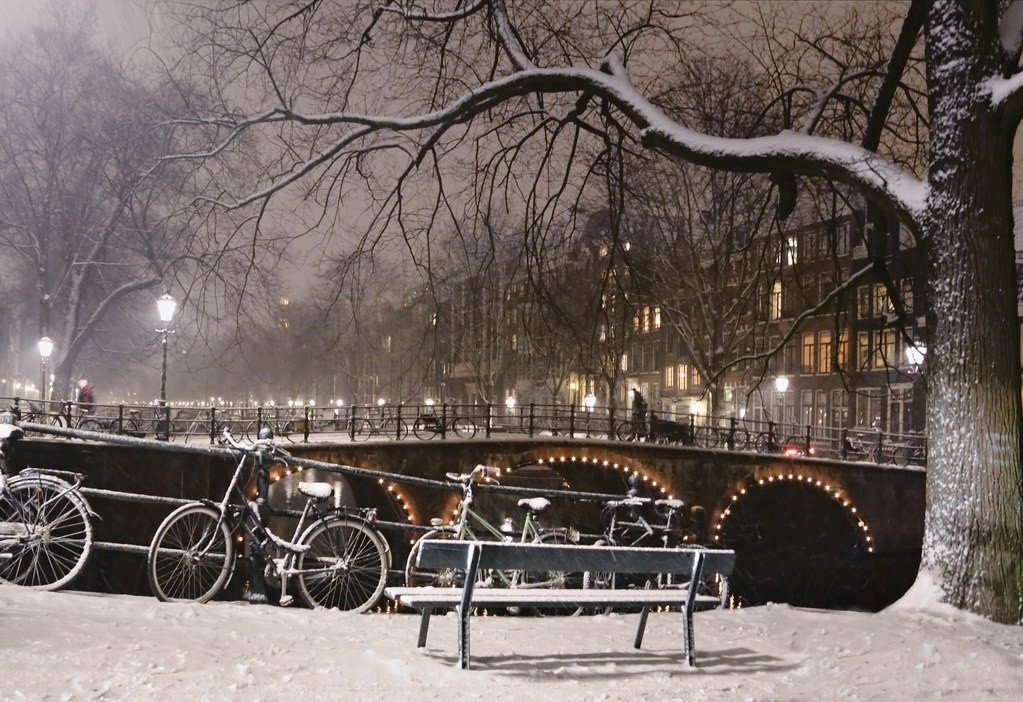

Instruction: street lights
[156,294,176,441]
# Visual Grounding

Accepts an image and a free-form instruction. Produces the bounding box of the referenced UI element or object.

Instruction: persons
[77,379,96,430]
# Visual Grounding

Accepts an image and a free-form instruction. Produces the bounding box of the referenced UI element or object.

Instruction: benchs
[384,540,736,669]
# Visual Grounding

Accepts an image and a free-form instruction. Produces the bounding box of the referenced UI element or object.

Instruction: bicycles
[413,399,476,440]
[694,415,751,451]
[37,336,53,412]
[755,424,808,456]
[246,407,310,444]
[22,400,104,434]
[0,423,103,592]
[523,409,571,437]
[891,430,927,468]
[405,464,585,620]
[147,427,394,615]
[185,405,244,446]
[110,405,177,443]
[585,409,679,446]
[582,490,732,617]
[843,427,895,465]
[347,402,408,441]
